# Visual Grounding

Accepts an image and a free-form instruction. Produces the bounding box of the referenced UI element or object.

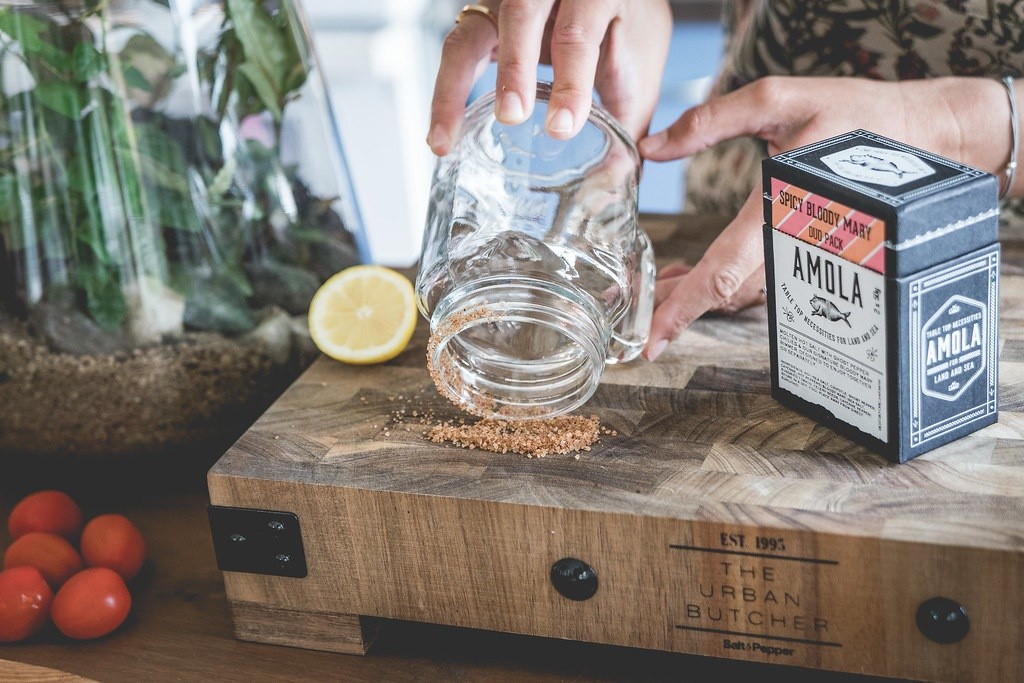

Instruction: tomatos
[0,490,145,644]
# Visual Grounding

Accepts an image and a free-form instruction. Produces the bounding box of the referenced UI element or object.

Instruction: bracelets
[1000,74,1019,198]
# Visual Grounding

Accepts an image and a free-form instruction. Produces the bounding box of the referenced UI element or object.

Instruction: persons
[427,0,1024,364]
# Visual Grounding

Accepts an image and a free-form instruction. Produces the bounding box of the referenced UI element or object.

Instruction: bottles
[413,78,657,422]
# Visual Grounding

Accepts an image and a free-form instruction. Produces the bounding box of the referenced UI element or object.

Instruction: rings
[456,5,500,35]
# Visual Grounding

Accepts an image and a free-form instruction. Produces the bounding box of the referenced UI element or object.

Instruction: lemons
[308,265,419,365]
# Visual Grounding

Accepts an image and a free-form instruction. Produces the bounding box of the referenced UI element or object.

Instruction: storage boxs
[761,128,1002,464]
